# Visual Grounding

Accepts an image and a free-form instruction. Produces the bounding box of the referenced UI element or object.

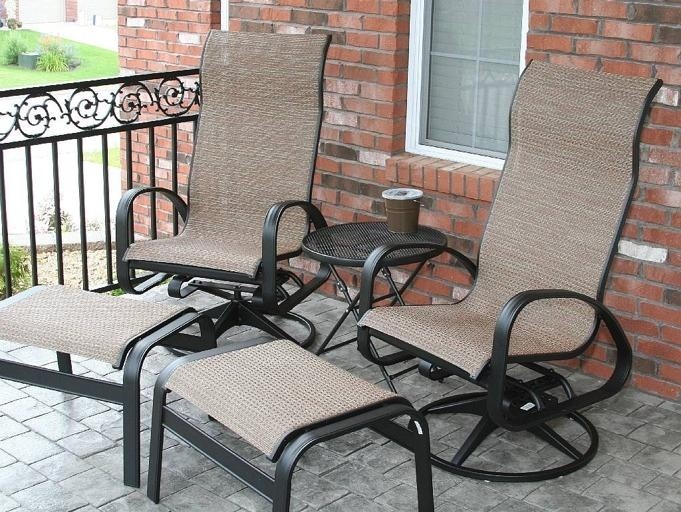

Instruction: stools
[0,283,216,488]
[147,335,436,511]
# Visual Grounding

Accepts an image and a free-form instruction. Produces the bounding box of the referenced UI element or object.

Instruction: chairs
[358,59,665,481]
[113,28,333,357]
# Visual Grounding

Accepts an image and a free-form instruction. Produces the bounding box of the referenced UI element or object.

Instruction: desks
[306,219,446,394]
